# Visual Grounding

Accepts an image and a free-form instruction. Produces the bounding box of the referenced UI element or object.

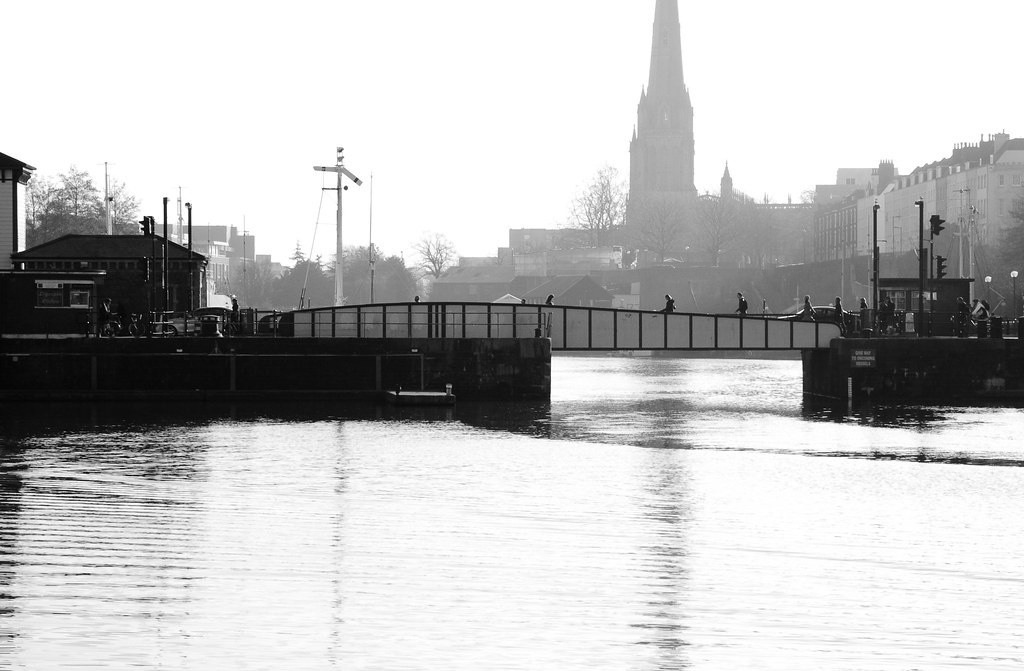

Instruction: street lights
[985,275,993,316]
[873,206,879,309]
[1009,270,1018,319]
[313,147,362,307]
[915,201,923,338]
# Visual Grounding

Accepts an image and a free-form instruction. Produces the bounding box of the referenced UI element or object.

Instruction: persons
[860,293,991,334]
[802,295,817,321]
[546,294,555,304]
[835,296,846,334]
[98,298,118,338]
[736,292,747,314]
[660,294,674,312]
[230,299,239,334]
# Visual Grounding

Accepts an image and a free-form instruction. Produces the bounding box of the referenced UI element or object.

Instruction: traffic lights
[138,215,151,243]
[936,256,947,279]
[933,215,945,235]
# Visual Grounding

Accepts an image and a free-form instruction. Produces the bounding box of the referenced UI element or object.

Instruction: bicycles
[99,312,123,337]
[129,314,155,337]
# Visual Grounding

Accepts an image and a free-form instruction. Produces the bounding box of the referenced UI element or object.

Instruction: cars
[155,314,202,335]
